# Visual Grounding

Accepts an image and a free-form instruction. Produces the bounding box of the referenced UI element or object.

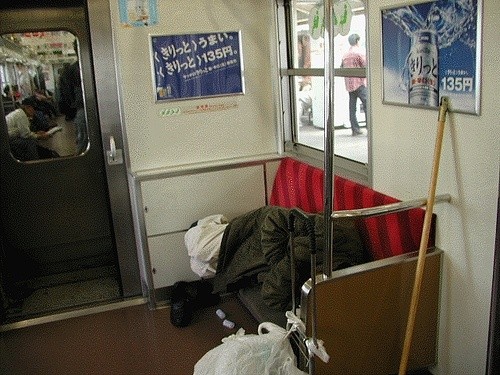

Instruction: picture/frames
[378,0,482,117]
[148,29,246,104]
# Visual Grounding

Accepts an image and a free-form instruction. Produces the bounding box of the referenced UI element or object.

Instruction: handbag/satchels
[191,309,329,374]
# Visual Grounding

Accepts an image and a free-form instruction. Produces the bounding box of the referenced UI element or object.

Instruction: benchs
[236,156,437,345]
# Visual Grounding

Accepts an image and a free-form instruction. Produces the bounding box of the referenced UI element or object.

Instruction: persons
[4,36,87,160]
[338,34,368,136]
